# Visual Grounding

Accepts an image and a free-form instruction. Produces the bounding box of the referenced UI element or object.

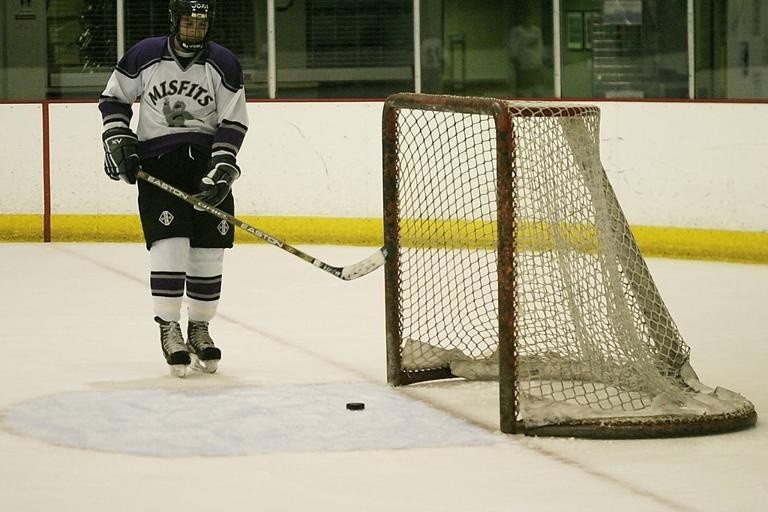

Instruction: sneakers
[188,317,221,361]
[155,316,191,366]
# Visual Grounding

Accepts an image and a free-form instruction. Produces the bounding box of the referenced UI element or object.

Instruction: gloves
[102,128,152,185]
[192,159,240,213]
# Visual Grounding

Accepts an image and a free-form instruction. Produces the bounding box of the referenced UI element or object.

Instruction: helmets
[168,1,216,52]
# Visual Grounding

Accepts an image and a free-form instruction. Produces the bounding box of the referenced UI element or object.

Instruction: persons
[420,24,448,95]
[93,0,251,364]
[507,6,552,101]
